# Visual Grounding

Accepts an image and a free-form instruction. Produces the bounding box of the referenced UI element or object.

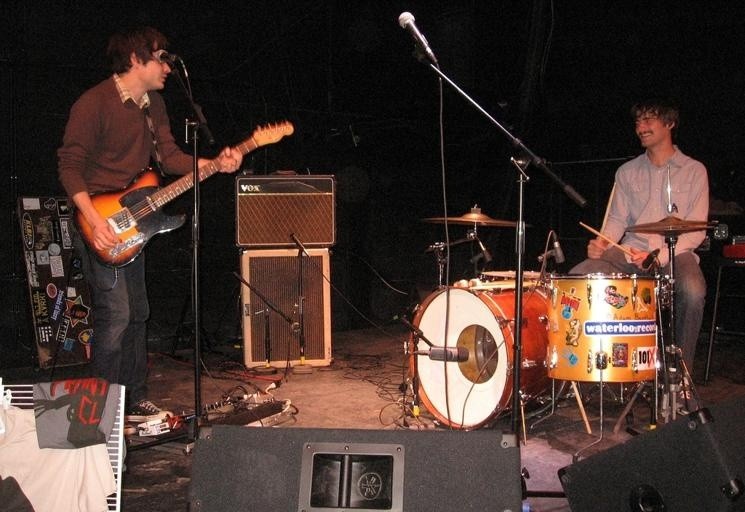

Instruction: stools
[699,239,745,388]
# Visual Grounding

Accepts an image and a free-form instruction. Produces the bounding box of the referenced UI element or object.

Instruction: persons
[56,23,242,424]
[566,92,710,416]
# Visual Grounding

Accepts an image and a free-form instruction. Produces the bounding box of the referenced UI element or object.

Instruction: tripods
[613,237,703,432]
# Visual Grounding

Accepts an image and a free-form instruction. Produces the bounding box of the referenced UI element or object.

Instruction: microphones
[475,236,496,270]
[399,12,438,64]
[550,231,566,264]
[642,248,660,268]
[430,346,469,361]
[291,233,312,258]
[155,49,177,63]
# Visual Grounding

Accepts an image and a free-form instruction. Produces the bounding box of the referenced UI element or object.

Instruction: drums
[482,269,551,281]
[548,274,659,382]
[407,280,568,429]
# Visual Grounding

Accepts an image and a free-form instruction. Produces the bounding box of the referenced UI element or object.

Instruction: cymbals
[421,214,523,227]
[628,218,710,235]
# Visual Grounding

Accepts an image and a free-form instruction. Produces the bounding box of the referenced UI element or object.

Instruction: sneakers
[122,419,138,436]
[669,376,702,419]
[124,398,177,422]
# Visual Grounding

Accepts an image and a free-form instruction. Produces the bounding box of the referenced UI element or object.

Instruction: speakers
[190,426,522,512]
[237,175,337,248]
[240,248,332,368]
[558,394,745,512]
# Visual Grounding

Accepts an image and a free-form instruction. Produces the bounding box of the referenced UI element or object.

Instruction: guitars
[72,121,294,267]
